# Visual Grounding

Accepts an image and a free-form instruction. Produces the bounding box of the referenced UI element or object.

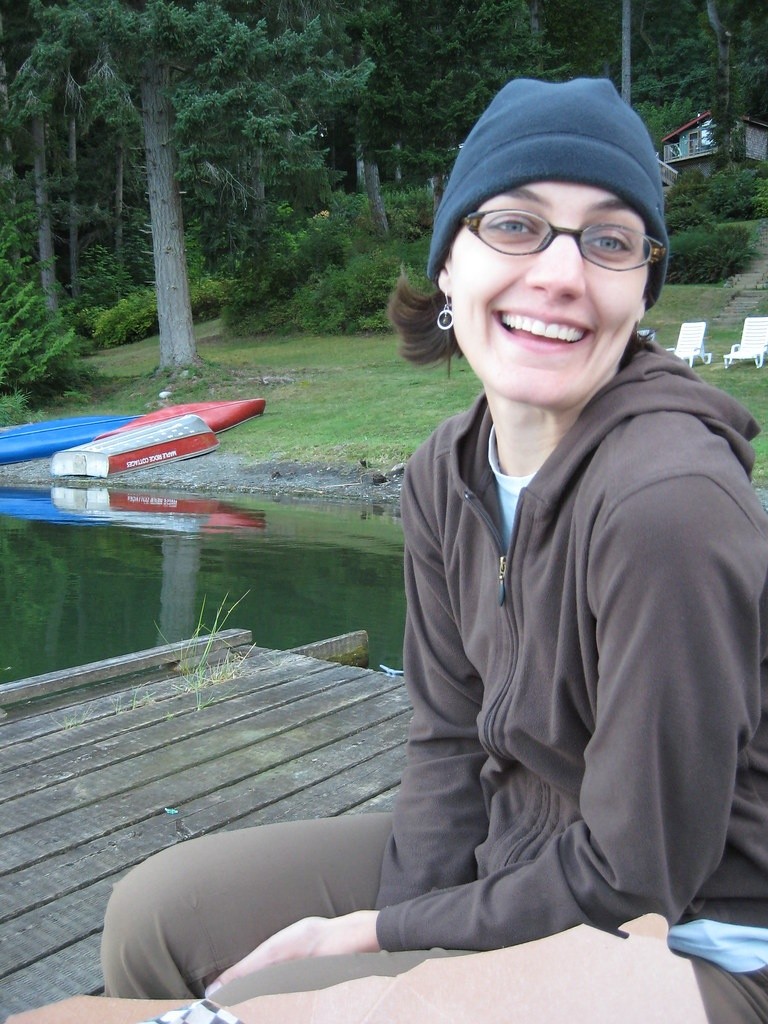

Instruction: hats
[426,79,671,310]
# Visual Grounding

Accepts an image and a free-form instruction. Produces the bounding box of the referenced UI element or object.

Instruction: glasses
[458,208,666,272]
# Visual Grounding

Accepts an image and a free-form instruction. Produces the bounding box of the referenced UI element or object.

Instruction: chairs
[723,315,768,368]
[663,321,712,367]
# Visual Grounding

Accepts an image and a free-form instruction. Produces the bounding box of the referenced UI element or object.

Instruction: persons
[101,78,768,1024]
